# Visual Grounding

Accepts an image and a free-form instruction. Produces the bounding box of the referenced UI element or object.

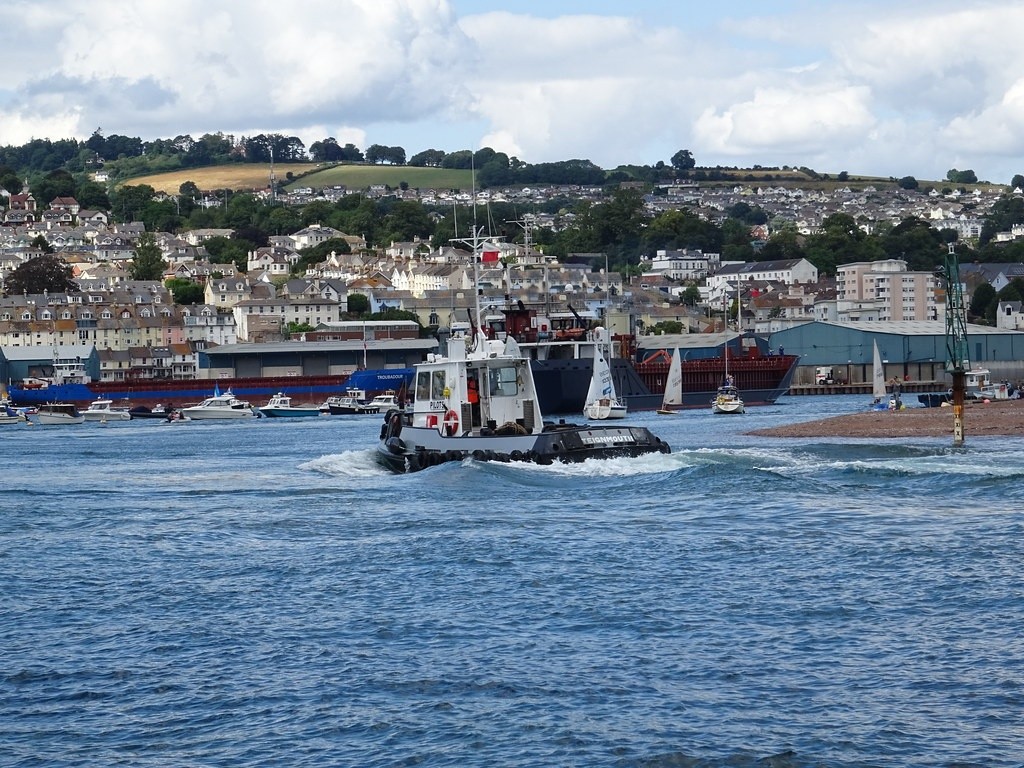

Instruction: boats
[368,390,400,413]
[257,392,320,417]
[182,390,261,420]
[919,369,1008,410]
[318,387,366,411]
[708,375,744,414]
[0,393,191,425]
[328,397,379,415]
[375,224,670,477]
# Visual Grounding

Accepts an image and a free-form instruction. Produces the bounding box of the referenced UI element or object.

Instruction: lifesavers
[444,409,458,434]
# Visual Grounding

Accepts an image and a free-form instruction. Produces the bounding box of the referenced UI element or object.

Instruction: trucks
[816,367,847,385]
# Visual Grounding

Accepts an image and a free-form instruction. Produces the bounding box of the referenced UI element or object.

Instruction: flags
[482,241,502,262]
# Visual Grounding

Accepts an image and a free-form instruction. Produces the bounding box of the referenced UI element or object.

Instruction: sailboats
[871,338,890,410]
[656,344,684,414]
[583,344,627,421]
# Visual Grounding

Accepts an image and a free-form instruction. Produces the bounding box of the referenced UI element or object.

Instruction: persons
[467,378,479,425]
[167,409,185,423]
[887,376,900,410]
